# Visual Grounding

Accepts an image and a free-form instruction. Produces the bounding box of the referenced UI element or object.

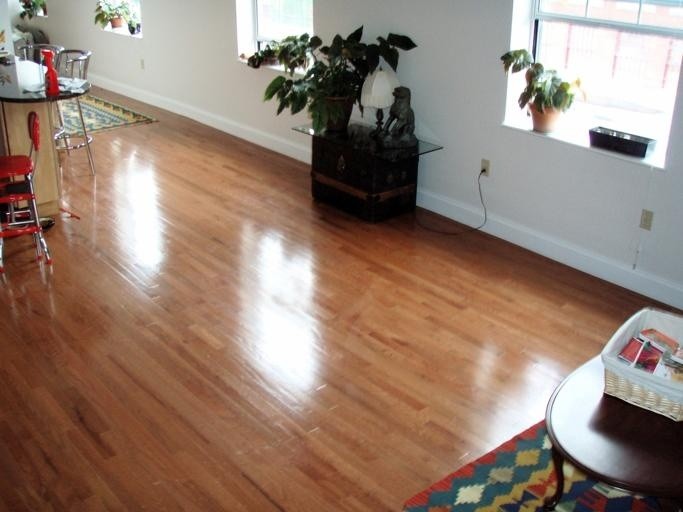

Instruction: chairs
[16,43,95,177]
[0,111,55,273]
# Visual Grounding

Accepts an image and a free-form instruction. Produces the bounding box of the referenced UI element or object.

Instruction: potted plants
[18,0,47,21]
[94,1,131,31]
[236,26,418,136]
[501,45,583,133]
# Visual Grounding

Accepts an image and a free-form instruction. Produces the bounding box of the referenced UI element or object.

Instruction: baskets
[602,306,683,423]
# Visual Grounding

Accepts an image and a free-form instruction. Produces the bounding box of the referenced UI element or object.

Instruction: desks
[0,79,92,217]
[531,354,681,512]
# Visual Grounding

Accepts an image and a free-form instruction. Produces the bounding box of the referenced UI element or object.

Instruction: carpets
[54,93,160,137]
[396,405,682,512]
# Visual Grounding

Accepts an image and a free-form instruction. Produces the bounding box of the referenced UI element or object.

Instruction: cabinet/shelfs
[291,121,443,224]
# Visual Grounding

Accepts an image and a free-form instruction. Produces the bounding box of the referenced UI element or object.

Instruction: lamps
[358,66,403,142]
